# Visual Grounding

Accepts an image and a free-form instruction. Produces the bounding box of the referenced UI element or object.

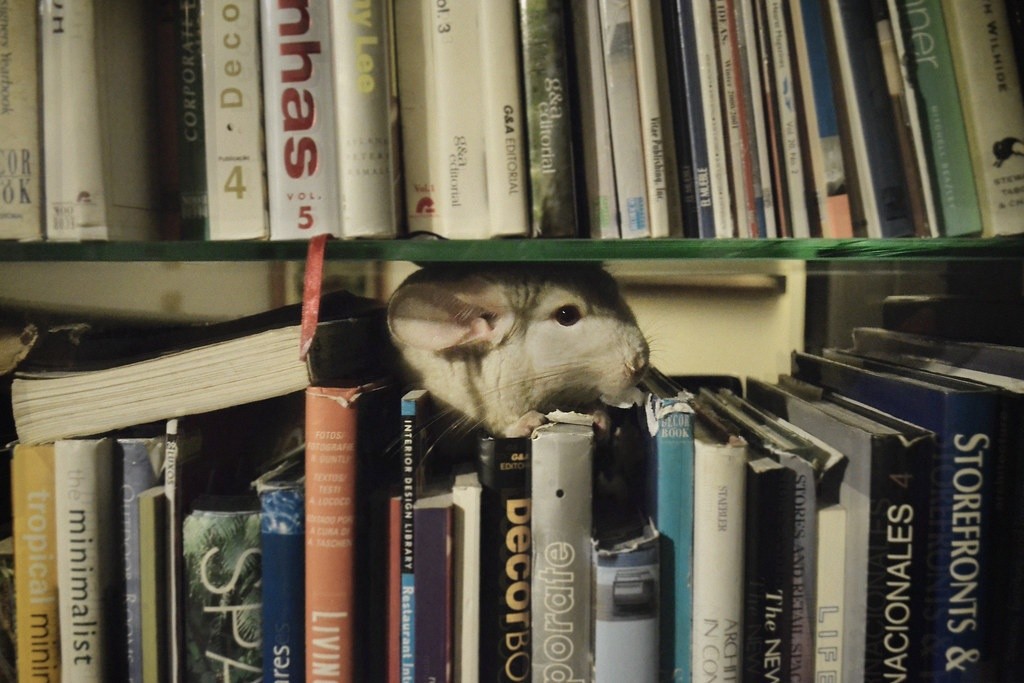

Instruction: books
[0,0,1024,242]
[0,291,1024,683]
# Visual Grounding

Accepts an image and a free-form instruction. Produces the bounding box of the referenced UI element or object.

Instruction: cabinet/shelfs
[0,0,1024,683]
